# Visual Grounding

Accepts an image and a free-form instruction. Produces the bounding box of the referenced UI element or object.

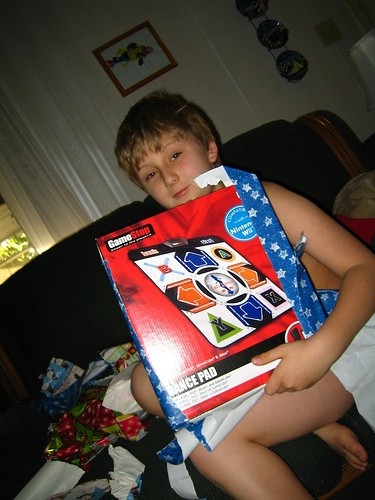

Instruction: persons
[114,89,375,500]
